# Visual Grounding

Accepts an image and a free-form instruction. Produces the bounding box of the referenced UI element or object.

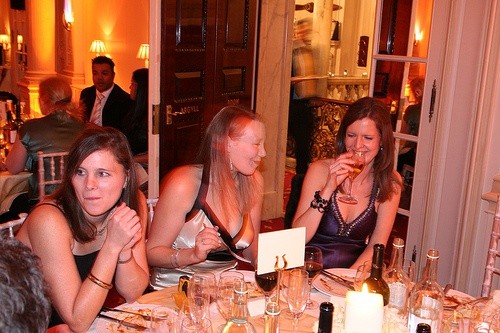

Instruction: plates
[96,303,179,333]
[311,267,371,298]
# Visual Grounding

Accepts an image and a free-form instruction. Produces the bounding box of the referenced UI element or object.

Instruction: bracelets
[311,192,330,212]
[171,248,180,267]
[117,255,134,264]
[175,249,185,268]
[87,274,113,289]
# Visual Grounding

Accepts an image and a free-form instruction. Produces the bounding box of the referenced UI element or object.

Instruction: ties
[91,94,105,127]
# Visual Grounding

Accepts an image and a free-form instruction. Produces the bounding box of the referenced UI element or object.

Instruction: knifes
[97,314,149,331]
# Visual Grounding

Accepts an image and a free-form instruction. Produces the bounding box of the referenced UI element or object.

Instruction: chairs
[1,151,69,239]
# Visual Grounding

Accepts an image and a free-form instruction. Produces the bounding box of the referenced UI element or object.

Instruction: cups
[150,306,178,333]
[468,300,500,333]
[178,270,215,333]
[438,308,465,333]
[216,321,257,333]
[216,270,244,320]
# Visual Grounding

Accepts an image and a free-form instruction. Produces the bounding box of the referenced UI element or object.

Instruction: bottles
[416,323,431,333]
[221,281,250,333]
[318,301,334,333]
[382,238,411,333]
[407,249,445,333]
[361,243,390,333]
[265,302,280,333]
[0,100,29,158]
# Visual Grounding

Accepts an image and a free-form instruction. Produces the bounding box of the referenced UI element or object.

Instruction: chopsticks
[320,269,354,291]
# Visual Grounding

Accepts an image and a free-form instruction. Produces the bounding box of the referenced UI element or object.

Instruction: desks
[0,171,32,211]
[85,272,483,333]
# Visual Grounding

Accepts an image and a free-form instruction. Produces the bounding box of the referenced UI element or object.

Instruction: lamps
[137,44,150,67]
[90,40,106,57]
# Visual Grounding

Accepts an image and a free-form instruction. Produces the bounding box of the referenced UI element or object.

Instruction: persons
[146,105,264,291]
[13,128,150,332]
[0,236,51,333]
[291,97,406,270]
[122,68,149,173]
[396,77,424,185]
[0,78,86,224]
[79,56,137,153]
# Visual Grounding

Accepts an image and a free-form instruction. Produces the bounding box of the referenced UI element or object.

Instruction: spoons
[202,223,252,263]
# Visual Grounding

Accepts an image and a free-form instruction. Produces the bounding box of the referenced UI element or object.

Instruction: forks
[101,306,167,321]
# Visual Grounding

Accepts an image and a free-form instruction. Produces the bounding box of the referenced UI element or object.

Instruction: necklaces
[96,206,117,235]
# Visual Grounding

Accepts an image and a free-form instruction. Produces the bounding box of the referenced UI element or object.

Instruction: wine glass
[254,260,278,319]
[338,148,366,205]
[305,245,323,310]
[287,269,309,333]
